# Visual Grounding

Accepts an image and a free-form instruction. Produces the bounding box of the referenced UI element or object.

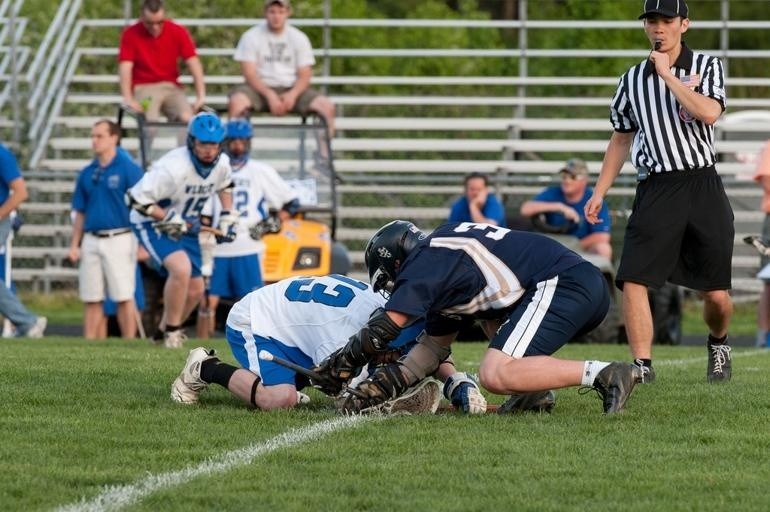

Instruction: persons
[0,144,48,339]
[520,164,614,272]
[308,218,640,415]
[172,273,488,416]
[68,115,300,349]
[583,0,734,384]
[117,0,209,123]
[451,171,507,228]
[225,0,336,137]
[742,142,770,348]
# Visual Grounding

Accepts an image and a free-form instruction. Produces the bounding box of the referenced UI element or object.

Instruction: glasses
[92,167,101,184]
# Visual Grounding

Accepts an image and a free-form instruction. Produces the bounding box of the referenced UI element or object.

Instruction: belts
[92,229,131,237]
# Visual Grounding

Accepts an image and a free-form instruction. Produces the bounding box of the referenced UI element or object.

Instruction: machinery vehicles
[108,106,352,338]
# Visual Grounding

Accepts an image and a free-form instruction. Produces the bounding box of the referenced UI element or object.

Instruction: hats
[558,158,587,176]
[265,0,289,8]
[638,0,689,20]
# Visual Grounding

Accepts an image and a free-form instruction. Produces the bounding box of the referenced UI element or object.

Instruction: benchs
[2,1,769,296]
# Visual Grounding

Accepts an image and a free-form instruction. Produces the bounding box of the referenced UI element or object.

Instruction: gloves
[214,211,239,244]
[309,347,364,399]
[443,371,488,416]
[161,207,190,236]
[342,361,408,418]
[249,214,280,241]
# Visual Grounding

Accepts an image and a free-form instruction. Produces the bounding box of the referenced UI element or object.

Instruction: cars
[446,211,684,344]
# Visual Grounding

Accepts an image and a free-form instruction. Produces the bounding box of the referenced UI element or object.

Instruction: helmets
[364,219,428,302]
[224,120,252,140]
[186,111,225,144]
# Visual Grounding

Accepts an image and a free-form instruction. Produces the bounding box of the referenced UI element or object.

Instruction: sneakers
[578,361,641,419]
[497,389,557,415]
[26,316,47,339]
[632,359,655,384]
[164,329,188,349]
[707,334,732,384]
[170,346,220,405]
